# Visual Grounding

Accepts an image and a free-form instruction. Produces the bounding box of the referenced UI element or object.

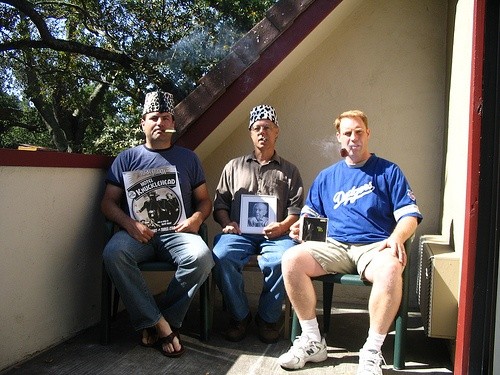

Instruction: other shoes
[226,317,253,342]
[254,316,279,343]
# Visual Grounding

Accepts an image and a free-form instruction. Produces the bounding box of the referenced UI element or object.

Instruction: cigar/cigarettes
[164,129,176,133]
[347,146,352,153]
[263,138,267,141]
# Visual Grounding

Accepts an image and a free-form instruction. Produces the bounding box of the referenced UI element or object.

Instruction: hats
[141,90,175,119]
[248,104,279,130]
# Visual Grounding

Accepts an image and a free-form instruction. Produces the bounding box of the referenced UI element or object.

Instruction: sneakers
[358,349,388,375]
[277,333,328,371]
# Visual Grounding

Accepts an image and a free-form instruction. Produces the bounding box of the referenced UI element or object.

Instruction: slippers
[158,323,185,357]
[138,326,160,347]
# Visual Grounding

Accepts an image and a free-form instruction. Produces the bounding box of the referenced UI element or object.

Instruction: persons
[248,202,269,227]
[212,104,305,344]
[100,91,215,358]
[278,109,423,375]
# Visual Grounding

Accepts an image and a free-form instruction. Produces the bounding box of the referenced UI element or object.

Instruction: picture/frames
[238,192,279,235]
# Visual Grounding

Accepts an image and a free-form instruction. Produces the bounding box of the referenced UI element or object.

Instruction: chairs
[97,219,413,371]
